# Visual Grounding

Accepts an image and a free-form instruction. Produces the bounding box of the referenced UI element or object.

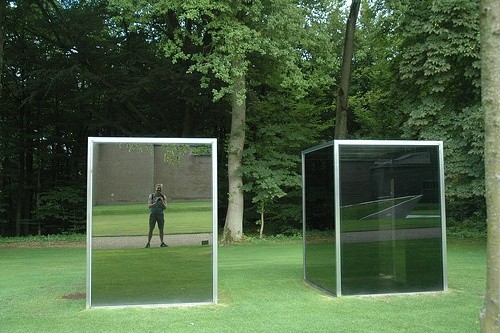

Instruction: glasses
[156,189,162,191]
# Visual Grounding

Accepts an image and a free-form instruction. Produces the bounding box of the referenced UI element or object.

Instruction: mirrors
[84,137,218,310]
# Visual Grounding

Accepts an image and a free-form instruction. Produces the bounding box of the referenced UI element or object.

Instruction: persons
[145,183,168,248]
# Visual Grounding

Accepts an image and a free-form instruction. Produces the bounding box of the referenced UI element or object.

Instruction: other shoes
[161,242,168,246]
[145,242,150,247]
